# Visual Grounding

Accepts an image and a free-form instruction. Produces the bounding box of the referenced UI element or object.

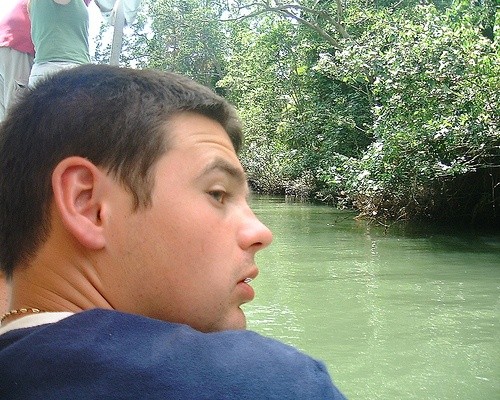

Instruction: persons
[0,0,36,126]
[27,0,91,93]
[2,61,351,400]
